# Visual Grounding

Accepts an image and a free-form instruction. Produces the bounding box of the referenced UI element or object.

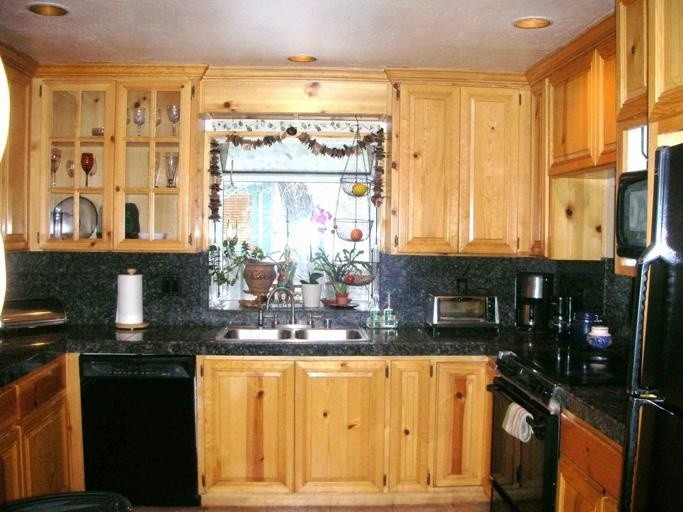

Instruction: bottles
[125,204,139,239]
[52,206,63,239]
[368,289,395,329]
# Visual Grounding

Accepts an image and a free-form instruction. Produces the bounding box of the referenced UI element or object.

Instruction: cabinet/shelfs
[530,12,616,176]
[29,63,202,254]
[646,0,683,123]
[0,354,71,504]
[435,355,486,505]
[556,409,624,512]
[196,355,389,508]
[615,0,647,122]
[385,69,541,256]
[0,42,38,252]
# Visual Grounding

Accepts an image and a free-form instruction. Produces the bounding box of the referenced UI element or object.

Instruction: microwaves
[424,292,504,327]
[616,168,655,269]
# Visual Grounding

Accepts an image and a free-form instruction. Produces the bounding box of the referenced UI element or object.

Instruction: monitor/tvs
[616,170,647,258]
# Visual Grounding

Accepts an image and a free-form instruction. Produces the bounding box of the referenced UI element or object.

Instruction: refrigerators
[616,144,682,512]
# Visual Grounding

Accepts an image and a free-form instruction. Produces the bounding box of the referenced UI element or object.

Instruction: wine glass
[144,152,178,186]
[133,104,179,138]
[47,148,96,190]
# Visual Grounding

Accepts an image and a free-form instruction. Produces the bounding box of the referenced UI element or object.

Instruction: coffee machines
[514,272,554,337]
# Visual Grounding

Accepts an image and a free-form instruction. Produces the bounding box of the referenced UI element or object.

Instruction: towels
[501,403,535,443]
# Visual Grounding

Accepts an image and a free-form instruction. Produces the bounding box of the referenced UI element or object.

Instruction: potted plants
[299,273,323,308]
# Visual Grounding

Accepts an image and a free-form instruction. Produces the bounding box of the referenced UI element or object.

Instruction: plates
[138,232,166,240]
[53,196,97,240]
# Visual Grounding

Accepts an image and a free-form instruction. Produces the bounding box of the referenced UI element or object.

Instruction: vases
[335,292,348,305]
[243,263,276,292]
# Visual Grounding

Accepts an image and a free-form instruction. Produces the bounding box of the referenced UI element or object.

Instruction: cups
[97,207,101,239]
[555,297,614,350]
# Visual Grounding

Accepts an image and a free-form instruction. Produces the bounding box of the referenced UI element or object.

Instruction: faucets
[266,287,296,325]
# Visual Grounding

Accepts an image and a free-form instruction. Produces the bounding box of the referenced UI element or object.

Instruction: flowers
[209,234,295,292]
[311,248,375,293]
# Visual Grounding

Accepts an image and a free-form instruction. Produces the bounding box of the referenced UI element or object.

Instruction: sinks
[295,326,370,345]
[214,325,291,344]
